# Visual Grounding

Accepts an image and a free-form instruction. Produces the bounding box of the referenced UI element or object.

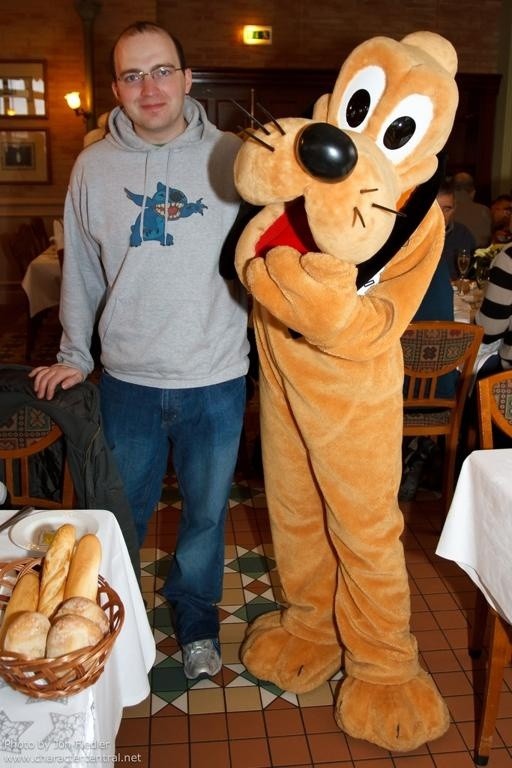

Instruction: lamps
[64,91,92,120]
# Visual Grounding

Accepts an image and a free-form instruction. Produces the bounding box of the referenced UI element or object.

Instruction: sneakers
[180,633,224,682]
[399,439,438,503]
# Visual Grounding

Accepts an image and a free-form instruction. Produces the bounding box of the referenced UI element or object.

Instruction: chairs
[469,370,512,659]
[0,364,77,510]
[400,321,485,516]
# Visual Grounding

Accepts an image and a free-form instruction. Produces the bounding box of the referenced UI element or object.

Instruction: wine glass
[456,249,473,280]
[474,259,489,304]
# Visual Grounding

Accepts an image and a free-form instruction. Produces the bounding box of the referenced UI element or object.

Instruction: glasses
[114,64,186,85]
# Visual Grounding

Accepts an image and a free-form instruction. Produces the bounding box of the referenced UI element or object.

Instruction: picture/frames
[1,127,53,185]
[0,59,49,120]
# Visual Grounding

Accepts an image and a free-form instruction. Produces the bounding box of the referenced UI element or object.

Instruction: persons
[397,170,511,504]
[28,22,249,681]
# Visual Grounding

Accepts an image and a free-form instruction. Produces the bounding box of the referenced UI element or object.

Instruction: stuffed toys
[223,28,458,756]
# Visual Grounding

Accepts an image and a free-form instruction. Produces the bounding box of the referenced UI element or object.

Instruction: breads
[62,533,102,605]
[2,573,40,640]
[38,523,75,616]
[53,596,111,636]
[4,611,51,669]
[46,613,104,682]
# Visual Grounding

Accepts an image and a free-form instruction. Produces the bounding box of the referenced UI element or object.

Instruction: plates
[451,280,473,292]
[8,509,101,555]
